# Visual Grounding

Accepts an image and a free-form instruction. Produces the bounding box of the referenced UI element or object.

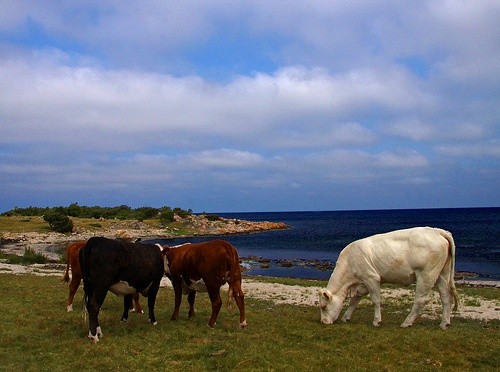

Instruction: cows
[163,239,249,331]
[317,226,458,332]
[63,236,145,315]
[79,234,165,345]
